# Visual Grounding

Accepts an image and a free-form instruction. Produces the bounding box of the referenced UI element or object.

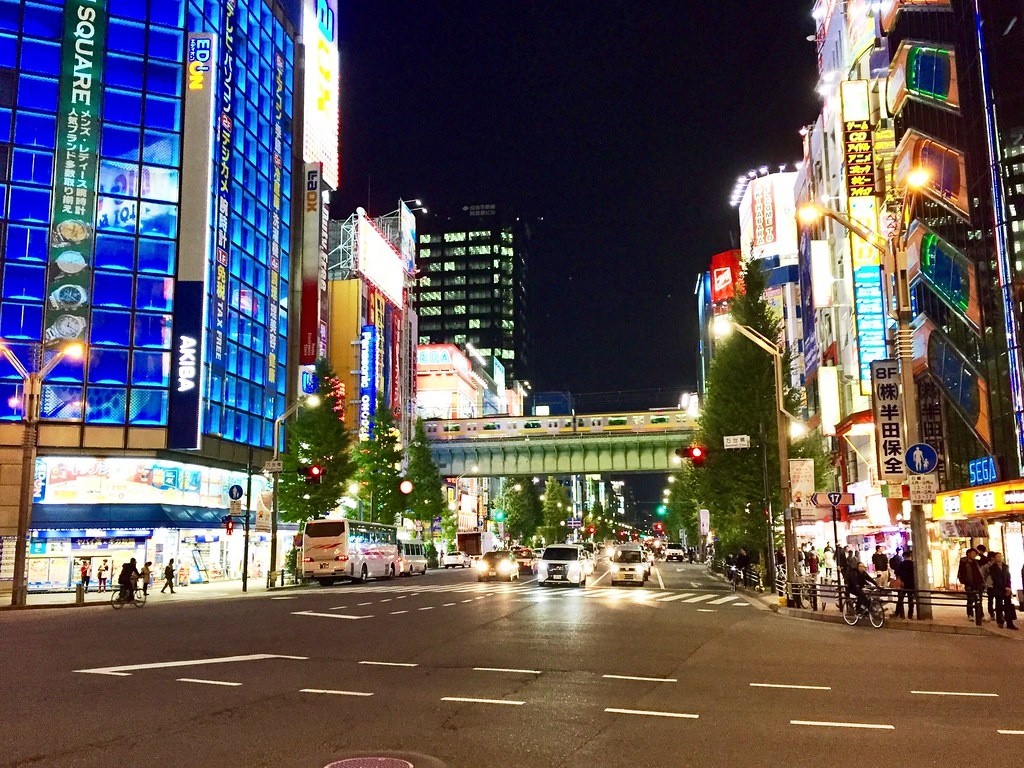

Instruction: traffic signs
[810,491,855,507]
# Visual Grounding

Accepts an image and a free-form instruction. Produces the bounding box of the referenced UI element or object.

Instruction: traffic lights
[298,466,326,477]
[496,512,504,521]
[691,448,704,467]
[312,476,322,484]
[227,520,233,532]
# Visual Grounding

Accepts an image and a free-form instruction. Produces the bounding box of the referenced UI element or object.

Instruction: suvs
[667,543,685,561]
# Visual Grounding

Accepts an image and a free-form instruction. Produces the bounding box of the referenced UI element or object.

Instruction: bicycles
[110,574,146,610]
[840,588,888,629]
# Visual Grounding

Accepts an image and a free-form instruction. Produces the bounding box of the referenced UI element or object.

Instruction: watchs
[51,219,92,248]
[50,250,90,283]
[45,313,87,342]
[47,284,90,312]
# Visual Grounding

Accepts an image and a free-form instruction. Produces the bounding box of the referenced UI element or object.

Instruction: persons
[81,561,91,594]
[833,544,860,584]
[797,541,819,574]
[118,558,145,602]
[872,545,889,587]
[160,558,176,593]
[958,544,1019,630]
[98,559,108,593]
[848,562,881,615]
[822,541,835,578]
[142,561,152,595]
[131,571,138,592]
[776,547,786,580]
[893,547,902,578]
[893,551,915,620]
[726,548,750,589]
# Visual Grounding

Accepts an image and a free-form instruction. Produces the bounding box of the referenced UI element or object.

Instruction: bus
[395,538,429,576]
[301,518,402,586]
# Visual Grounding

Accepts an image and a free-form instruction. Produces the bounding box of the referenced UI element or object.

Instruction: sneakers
[997,622,1003,627]
[968,615,975,621]
[1007,623,1018,629]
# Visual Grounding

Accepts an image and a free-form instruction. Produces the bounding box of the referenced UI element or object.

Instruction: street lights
[455,467,480,550]
[270,393,319,588]
[1,336,86,603]
[798,167,937,614]
[502,485,520,550]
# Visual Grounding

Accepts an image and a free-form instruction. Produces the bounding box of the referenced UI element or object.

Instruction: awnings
[28,503,302,530]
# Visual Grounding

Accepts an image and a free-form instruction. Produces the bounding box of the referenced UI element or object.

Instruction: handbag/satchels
[1003,603,1017,620]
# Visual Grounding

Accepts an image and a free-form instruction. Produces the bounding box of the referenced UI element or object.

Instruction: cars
[536,544,587,589]
[443,551,473,569]
[476,551,521,582]
[510,543,545,576]
[570,538,665,586]
